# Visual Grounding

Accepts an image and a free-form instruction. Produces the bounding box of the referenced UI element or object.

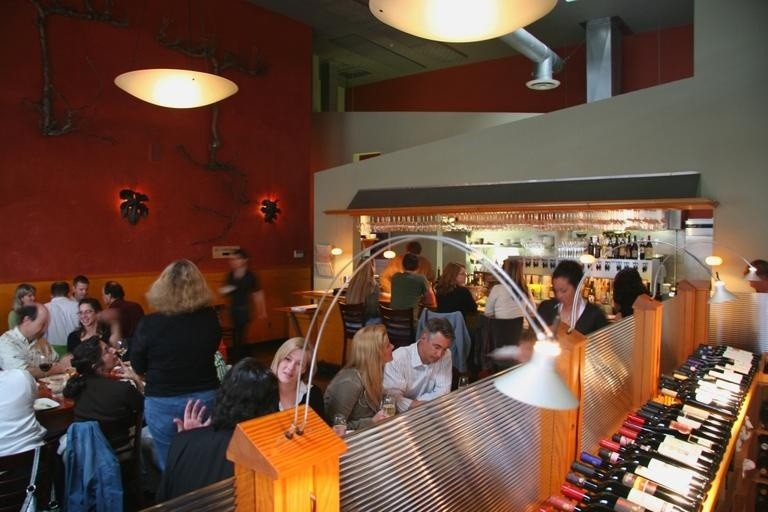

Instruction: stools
[273,306,321,377]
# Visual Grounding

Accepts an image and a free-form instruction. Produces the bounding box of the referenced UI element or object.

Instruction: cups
[50,376,65,397]
[556,240,586,258]
[332,414,348,437]
[458,375,468,389]
[383,396,396,417]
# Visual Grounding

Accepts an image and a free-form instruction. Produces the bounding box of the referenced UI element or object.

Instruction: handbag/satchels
[19,445,64,512]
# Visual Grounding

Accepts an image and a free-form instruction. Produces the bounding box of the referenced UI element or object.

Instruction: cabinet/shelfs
[294,287,351,365]
[701,351,767,512]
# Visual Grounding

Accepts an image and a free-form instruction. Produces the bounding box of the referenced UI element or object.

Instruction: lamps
[566,239,761,334]
[119,188,148,223]
[286,234,581,439]
[368,0,558,45]
[260,199,280,224]
[111,0,240,110]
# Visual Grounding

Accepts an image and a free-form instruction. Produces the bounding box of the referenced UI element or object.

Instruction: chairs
[0,409,142,512]
[337,301,486,387]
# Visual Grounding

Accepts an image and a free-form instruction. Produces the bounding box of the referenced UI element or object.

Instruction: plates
[33,398,60,410]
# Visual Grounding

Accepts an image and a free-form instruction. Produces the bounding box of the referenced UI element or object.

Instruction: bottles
[656,343,760,417]
[645,282,653,297]
[435,268,441,283]
[585,279,597,304]
[750,394,768,511]
[528,275,555,300]
[654,283,663,302]
[533,399,736,512]
[588,234,655,260]
[464,272,484,287]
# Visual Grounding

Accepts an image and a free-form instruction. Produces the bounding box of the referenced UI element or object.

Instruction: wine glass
[116,339,129,363]
[38,351,52,385]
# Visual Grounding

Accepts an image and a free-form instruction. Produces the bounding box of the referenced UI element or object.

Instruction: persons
[64,335,147,466]
[153,356,280,503]
[610,267,649,317]
[485,259,536,322]
[324,323,394,431]
[0,248,146,376]
[345,256,383,335]
[217,249,267,365]
[388,254,436,338]
[743,259,768,293]
[379,241,433,294]
[524,260,607,341]
[0,367,57,511]
[271,337,324,424]
[437,262,479,322]
[128,259,224,475]
[384,319,455,412]
